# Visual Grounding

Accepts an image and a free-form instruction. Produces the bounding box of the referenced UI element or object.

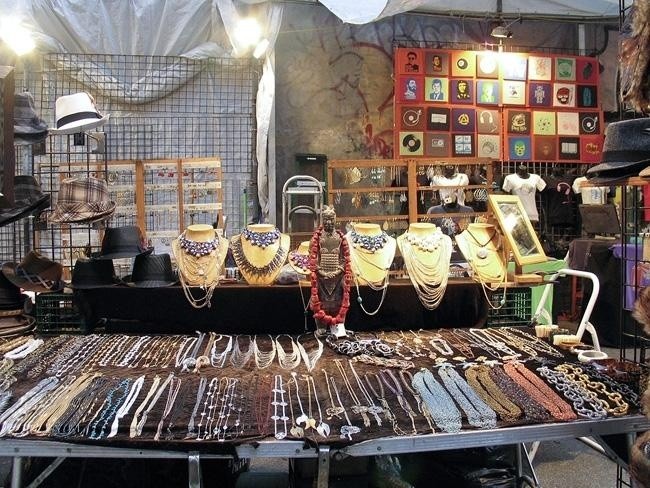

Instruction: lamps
[491,16,522,38]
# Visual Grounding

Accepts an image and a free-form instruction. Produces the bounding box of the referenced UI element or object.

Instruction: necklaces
[0,226,649,444]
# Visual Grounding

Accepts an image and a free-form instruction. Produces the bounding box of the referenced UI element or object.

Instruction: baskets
[37,294,88,336]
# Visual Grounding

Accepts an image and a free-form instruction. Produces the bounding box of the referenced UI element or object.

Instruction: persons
[404,79,417,101]
[430,79,443,100]
[556,87,570,104]
[502,165,548,223]
[456,80,471,100]
[432,55,443,72]
[404,51,419,72]
[426,195,474,260]
[431,166,469,205]
[535,83,544,104]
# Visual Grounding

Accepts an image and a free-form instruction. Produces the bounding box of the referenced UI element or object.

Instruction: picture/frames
[488,195,548,266]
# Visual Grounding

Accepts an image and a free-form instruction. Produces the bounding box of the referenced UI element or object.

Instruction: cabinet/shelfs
[327,157,494,224]
[282,175,323,235]
[58,157,224,280]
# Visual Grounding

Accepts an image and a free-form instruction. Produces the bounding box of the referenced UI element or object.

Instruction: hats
[49,178,116,225]
[122,254,180,289]
[47,93,110,135]
[65,258,123,289]
[2,251,65,293]
[0,175,50,227]
[92,226,153,261]
[13,92,49,146]
[586,118,650,183]
[1,270,37,337]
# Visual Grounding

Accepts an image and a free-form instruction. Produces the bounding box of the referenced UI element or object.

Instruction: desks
[0,327,649,487]
[68,277,545,330]
